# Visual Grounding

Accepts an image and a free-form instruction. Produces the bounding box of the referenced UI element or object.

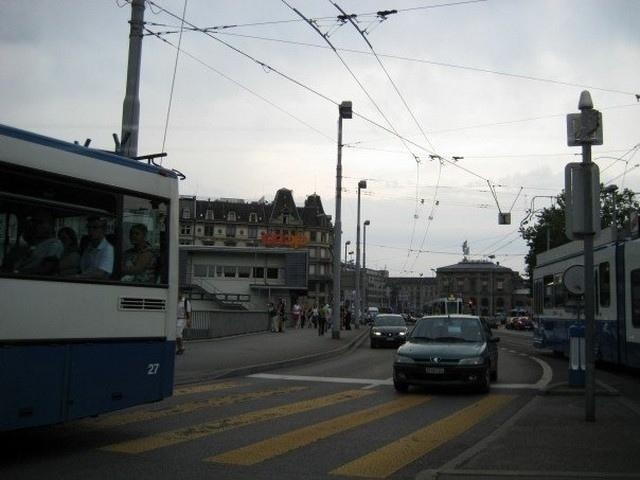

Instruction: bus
[0,122,181,435]
[423,293,465,314]
[531,223,639,374]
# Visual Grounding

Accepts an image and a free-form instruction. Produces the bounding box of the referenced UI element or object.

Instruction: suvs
[392,313,501,395]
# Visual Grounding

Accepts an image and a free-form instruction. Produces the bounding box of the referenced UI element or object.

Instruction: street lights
[333,96,353,342]
[607,185,620,226]
[344,180,371,328]
[542,222,554,251]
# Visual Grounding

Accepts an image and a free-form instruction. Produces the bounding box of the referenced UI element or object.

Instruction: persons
[175,287,193,355]
[266,295,333,337]
[1,206,160,285]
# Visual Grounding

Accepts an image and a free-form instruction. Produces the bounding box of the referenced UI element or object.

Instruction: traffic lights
[468,299,473,307]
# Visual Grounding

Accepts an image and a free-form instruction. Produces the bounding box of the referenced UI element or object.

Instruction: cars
[349,304,534,331]
[369,312,409,348]
